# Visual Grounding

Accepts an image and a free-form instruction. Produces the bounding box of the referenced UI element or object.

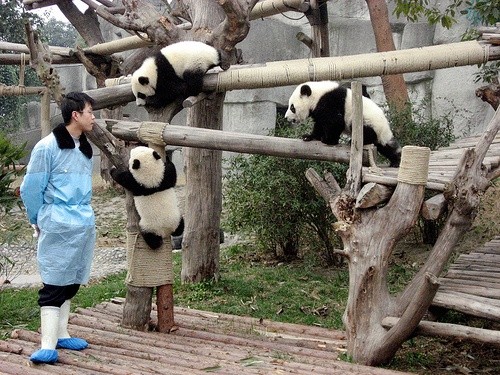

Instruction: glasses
[78,109,96,115]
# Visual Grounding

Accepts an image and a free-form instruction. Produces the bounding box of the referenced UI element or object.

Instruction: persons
[19,92,96,363]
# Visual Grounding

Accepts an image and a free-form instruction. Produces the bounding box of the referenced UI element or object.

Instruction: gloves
[31,223,40,238]
